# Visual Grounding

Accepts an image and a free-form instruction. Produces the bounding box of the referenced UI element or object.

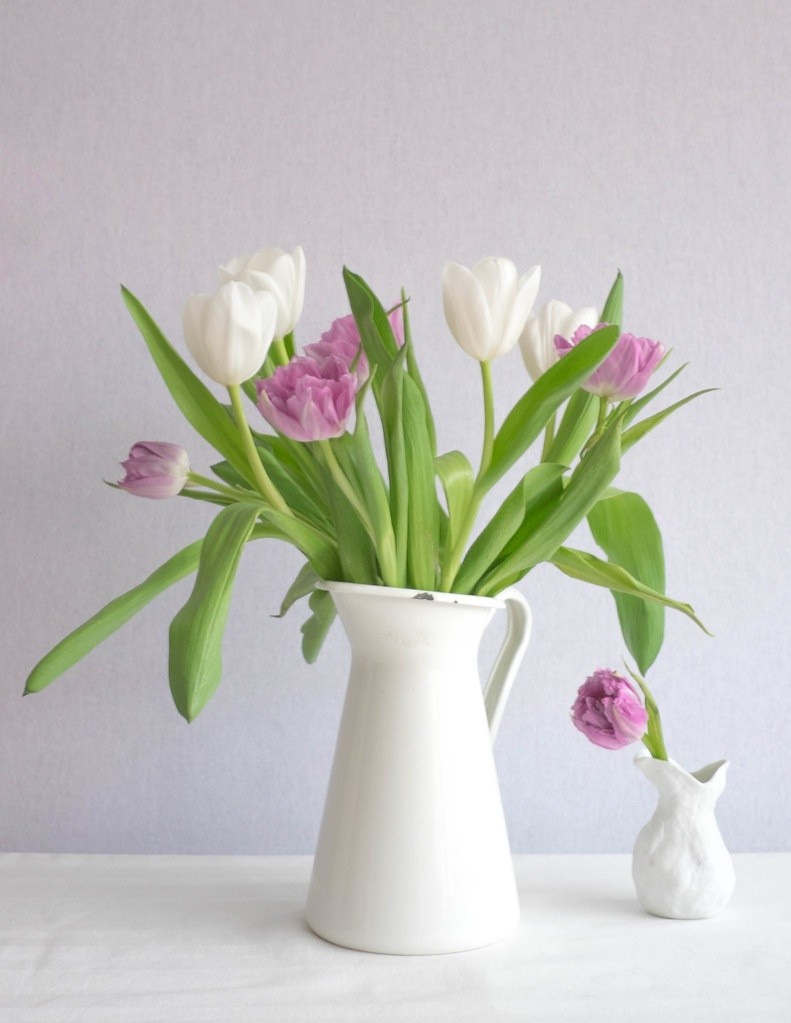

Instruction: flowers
[22,247,721,721]
[569,652,666,760]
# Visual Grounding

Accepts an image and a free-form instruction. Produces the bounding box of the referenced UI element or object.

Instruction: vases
[633,757,734,920]
[302,584,533,955]
